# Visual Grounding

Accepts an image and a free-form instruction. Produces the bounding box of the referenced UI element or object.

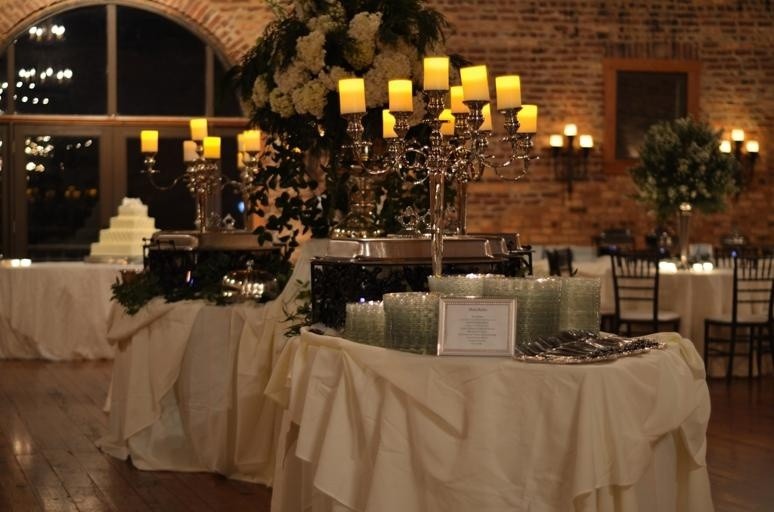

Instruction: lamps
[339,58,537,276]
[141,116,260,232]
[721,130,759,201]
[551,124,593,194]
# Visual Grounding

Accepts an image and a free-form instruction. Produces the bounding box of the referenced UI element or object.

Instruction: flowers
[623,110,741,215]
[233,0,455,265]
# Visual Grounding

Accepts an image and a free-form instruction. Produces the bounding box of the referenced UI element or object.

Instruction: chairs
[546,246,614,332]
[610,250,680,336]
[705,251,774,386]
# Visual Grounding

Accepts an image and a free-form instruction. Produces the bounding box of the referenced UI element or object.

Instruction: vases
[678,204,692,270]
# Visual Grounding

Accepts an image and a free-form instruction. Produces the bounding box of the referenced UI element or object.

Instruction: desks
[264,329,716,512]
[89,299,275,487]
[534,255,774,378]
[0,259,145,364]
